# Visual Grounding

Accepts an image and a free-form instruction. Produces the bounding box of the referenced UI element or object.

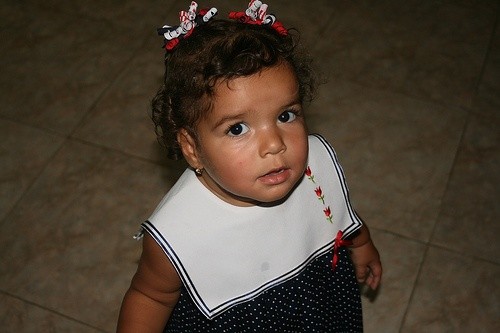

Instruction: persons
[116,0,384,333]
